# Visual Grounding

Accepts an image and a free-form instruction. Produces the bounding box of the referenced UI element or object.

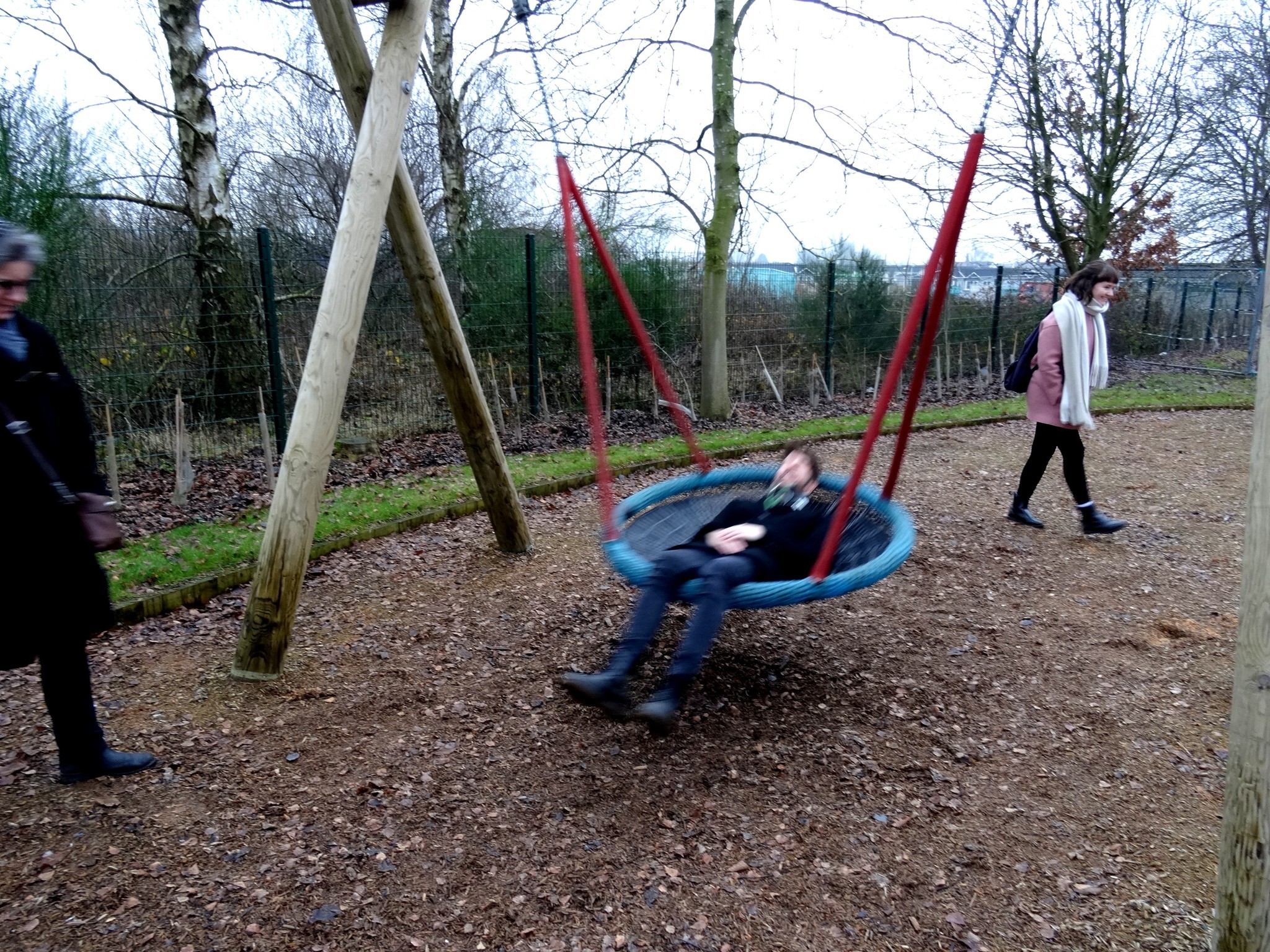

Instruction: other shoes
[59,744,158,785]
[631,696,682,739]
[558,668,622,719]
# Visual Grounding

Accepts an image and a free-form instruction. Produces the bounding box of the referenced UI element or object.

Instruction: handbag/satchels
[72,490,126,554]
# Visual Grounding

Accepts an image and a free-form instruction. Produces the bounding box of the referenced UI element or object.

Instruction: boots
[1008,491,1044,527]
[1075,499,1128,535]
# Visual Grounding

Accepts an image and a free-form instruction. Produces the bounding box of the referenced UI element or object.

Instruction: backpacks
[1003,324,1039,393]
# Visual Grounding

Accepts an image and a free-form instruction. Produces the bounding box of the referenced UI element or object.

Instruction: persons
[560,450,832,738]
[1008,260,1130,536]
[0,219,157,784]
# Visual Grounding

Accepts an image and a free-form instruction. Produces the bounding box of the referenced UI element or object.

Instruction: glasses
[0,278,34,290]
